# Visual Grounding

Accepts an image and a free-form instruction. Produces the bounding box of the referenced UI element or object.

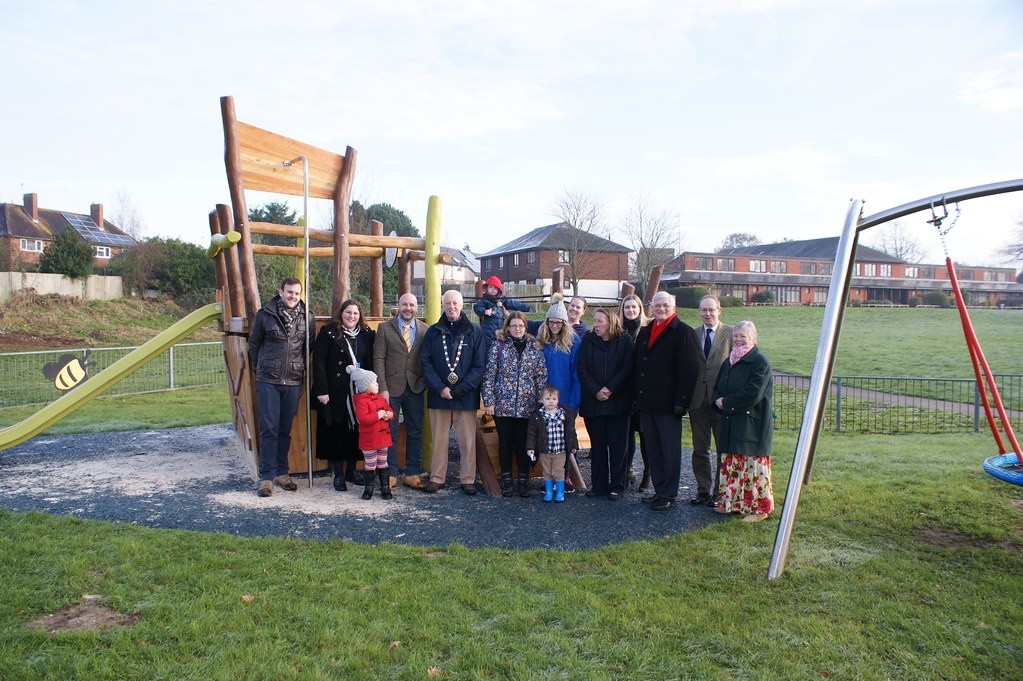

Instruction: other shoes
[713,507,729,514]
[740,512,767,523]
[258,469,531,501]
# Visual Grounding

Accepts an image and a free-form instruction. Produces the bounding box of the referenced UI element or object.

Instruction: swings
[924,197,1023,486]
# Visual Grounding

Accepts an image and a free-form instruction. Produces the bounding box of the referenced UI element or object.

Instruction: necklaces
[441,332,465,385]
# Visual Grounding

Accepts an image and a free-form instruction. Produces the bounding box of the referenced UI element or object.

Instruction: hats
[545,293,568,323]
[346,365,378,393]
[482,276,503,297]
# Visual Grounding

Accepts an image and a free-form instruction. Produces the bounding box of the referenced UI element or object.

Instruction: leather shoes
[651,498,676,511]
[708,496,719,507]
[642,494,659,505]
[691,497,708,505]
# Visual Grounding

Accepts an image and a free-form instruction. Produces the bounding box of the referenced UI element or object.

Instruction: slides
[0,300,224,452]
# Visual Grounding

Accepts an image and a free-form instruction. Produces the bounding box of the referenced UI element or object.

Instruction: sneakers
[608,492,621,500]
[585,490,602,497]
[540,482,556,493]
[564,478,575,494]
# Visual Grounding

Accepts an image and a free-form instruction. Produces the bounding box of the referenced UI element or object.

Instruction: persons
[480,311,549,497]
[248,277,315,497]
[526,387,579,503]
[613,295,653,492]
[711,320,776,522]
[575,307,633,501]
[372,293,431,491]
[344,365,396,501]
[686,295,737,507]
[632,290,702,512]
[474,276,530,358]
[310,300,376,493]
[500,294,589,461]
[535,292,581,493]
[419,290,485,495]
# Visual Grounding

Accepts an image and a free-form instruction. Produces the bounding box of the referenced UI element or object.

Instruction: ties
[403,325,412,353]
[704,329,713,360]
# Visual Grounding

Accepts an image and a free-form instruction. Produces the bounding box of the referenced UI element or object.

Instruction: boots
[624,472,633,492]
[543,479,553,502]
[555,480,565,503]
[639,470,650,491]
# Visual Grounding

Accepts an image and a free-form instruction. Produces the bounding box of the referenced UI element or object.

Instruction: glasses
[652,304,673,310]
[509,324,525,329]
[547,320,564,326]
[698,308,717,314]
